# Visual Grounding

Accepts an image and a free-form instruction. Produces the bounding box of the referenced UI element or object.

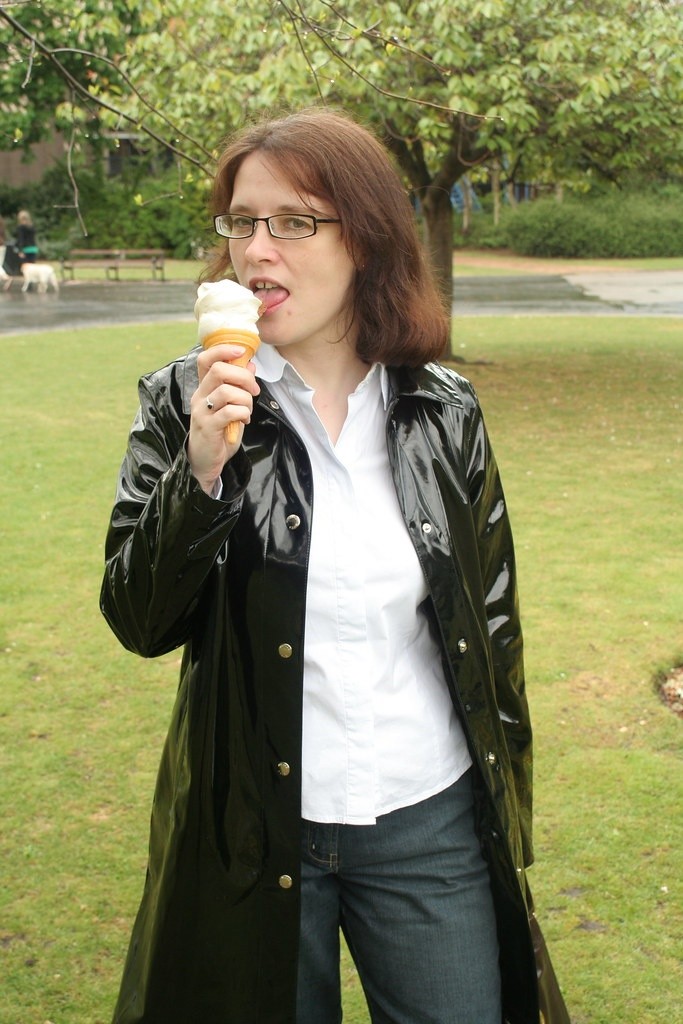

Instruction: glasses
[213,213,341,240]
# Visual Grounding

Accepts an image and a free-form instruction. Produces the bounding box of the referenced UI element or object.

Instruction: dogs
[21,262,59,293]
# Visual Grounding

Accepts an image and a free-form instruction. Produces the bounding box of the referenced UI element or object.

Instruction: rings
[204,397,216,411]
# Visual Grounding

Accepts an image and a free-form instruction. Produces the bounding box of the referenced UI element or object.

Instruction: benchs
[61,247,165,283]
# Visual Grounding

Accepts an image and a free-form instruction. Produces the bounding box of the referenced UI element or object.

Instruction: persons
[98,109,578,1024]
[4,210,37,277]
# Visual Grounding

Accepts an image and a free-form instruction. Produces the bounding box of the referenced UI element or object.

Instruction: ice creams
[193,278,263,444]
